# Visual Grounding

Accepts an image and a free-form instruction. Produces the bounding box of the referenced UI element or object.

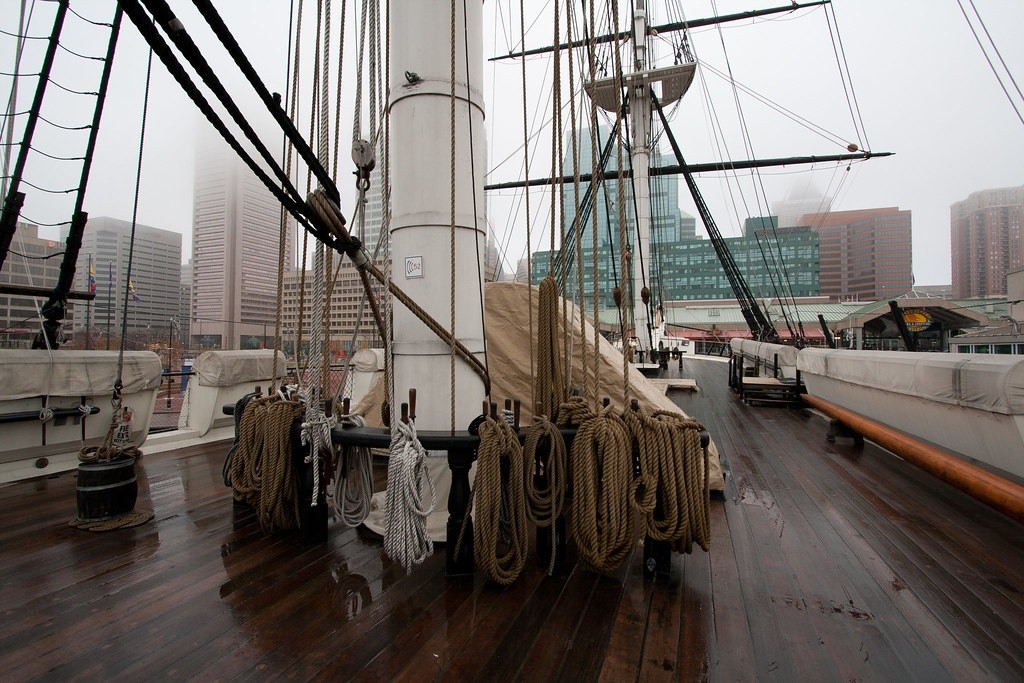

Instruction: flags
[89,255,97,296]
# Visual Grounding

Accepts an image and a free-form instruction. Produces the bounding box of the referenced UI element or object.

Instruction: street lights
[166,318,173,408]
